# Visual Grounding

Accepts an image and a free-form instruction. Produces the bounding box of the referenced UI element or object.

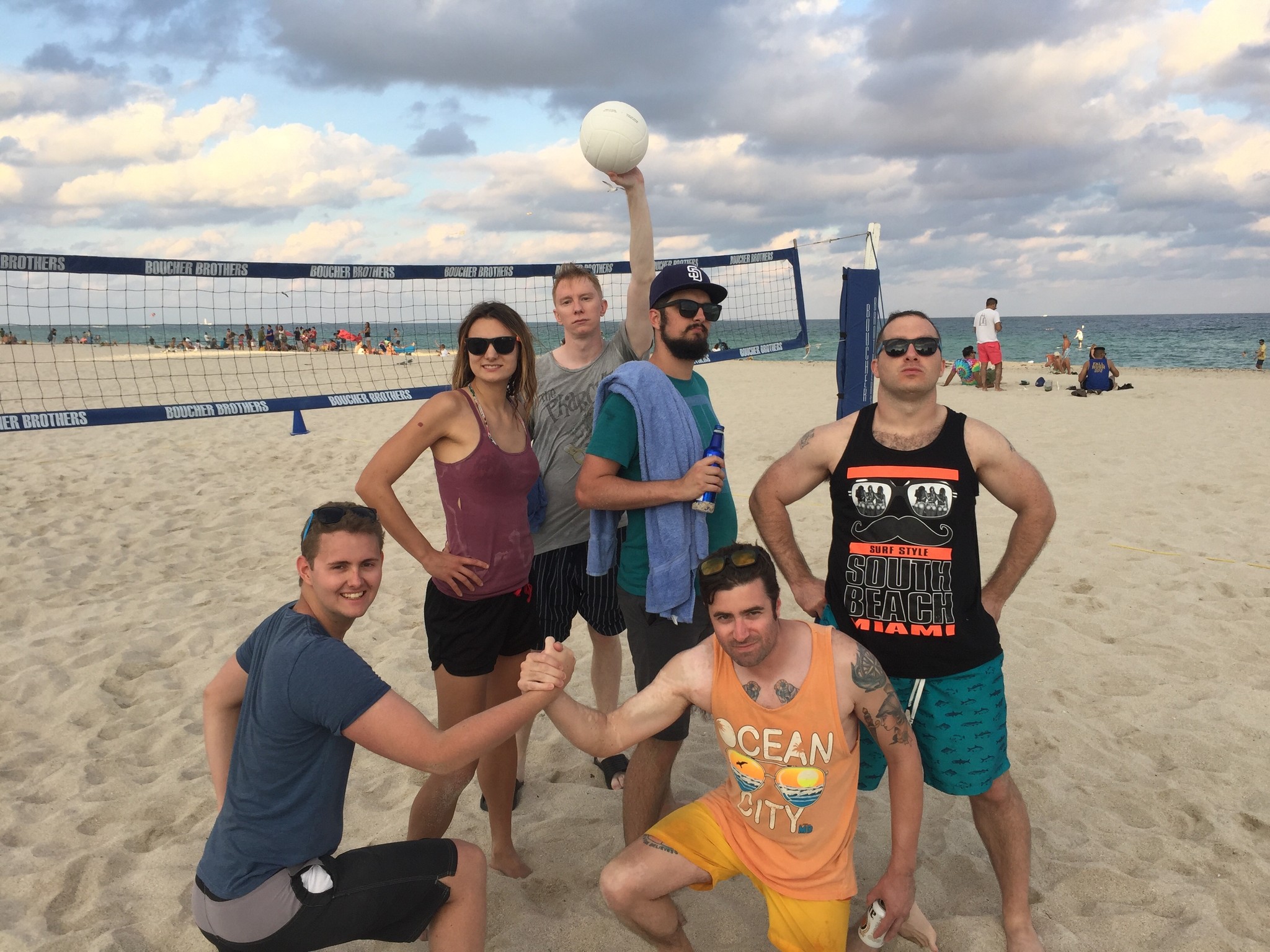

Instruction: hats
[1054,351,1060,357]
[648,264,728,310]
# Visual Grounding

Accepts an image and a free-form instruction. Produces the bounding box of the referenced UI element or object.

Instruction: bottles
[691,425,724,513]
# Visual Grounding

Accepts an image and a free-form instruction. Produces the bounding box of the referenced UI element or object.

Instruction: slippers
[480,778,524,811]
[593,754,629,790]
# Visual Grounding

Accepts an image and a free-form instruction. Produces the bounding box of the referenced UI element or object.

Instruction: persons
[1089,344,1096,360]
[1045,351,1071,375]
[354,300,542,879]
[940,346,1003,388]
[0,322,447,358]
[516,541,938,952]
[973,298,1006,391]
[480,164,654,812]
[1074,329,1083,348]
[1078,347,1119,392]
[1061,334,1071,358]
[746,311,1055,952]
[191,502,576,952]
[576,265,737,925]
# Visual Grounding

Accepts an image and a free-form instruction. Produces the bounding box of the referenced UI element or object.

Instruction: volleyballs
[578,99,648,174]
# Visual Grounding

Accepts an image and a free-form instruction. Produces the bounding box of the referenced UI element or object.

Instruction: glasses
[969,351,976,355]
[463,335,521,355]
[877,336,942,358]
[303,505,379,541]
[698,548,776,577]
[1101,352,1107,356]
[654,299,722,322]
